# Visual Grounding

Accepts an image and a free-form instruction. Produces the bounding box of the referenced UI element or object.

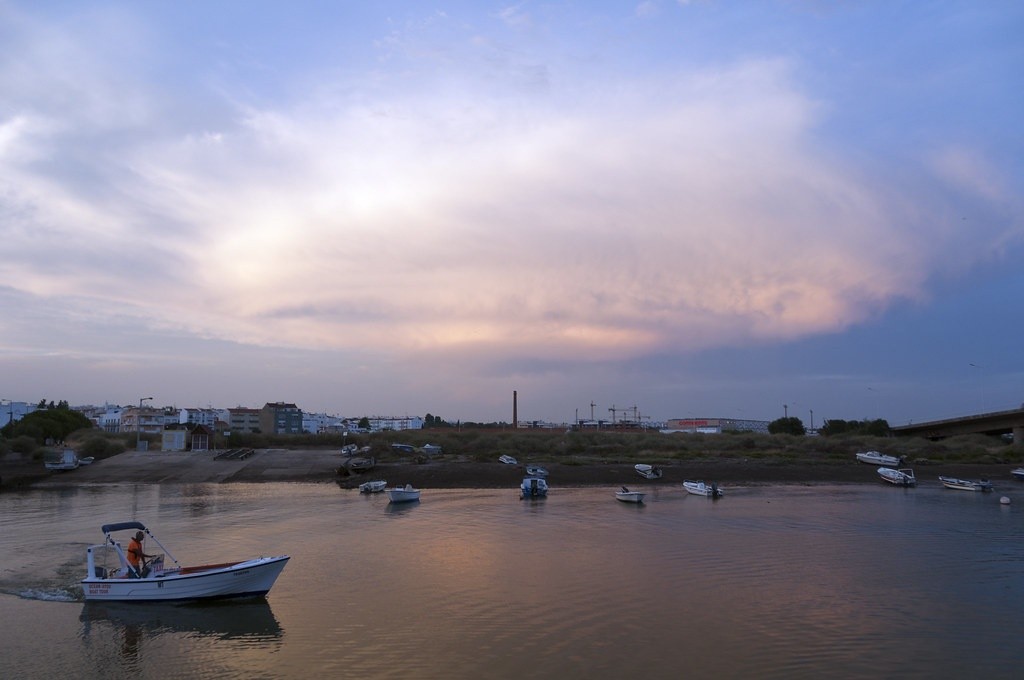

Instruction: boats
[635,463,664,480]
[393,444,414,453]
[876,465,917,487]
[856,450,902,468]
[938,474,995,492]
[683,478,724,497]
[81,521,291,604]
[499,455,518,465]
[383,483,421,504]
[341,443,376,474]
[520,475,548,498]
[525,466,551,480]
[44,439,81,471]
[359,480,388,494]
[423,444,442,455]
[1009,467,1024,481]
[615,486,647,503]
[78,456,95,466]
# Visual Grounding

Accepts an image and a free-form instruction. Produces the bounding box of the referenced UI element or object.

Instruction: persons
[127,531,154,578]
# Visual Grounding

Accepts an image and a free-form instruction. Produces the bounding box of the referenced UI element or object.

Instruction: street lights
[810,409,813,434]
[783,405,788,418]
[2,399,12,421]
[137,397,153,441]
